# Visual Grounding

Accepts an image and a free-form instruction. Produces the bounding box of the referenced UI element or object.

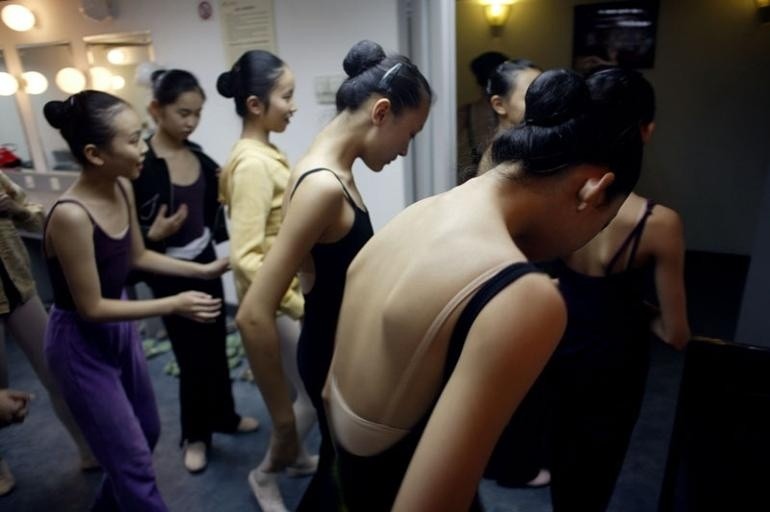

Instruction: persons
[295,68,647,508]
[127,67,263,476]
[218,48,319,511]
[43,89,234,510]
[0,167,100,494]
[0,383,34,427]
[236,40,433,509]
[473,50,552,489]
[552,68,692,507]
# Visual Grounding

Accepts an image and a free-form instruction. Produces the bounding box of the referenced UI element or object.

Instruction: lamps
[475,0,521,38]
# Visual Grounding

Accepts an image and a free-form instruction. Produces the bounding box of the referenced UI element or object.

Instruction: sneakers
[529,469,551,486]
[286,454,320,477]
[239,417,259,433]
[249,470,289,512]
[185,442,207,472]
